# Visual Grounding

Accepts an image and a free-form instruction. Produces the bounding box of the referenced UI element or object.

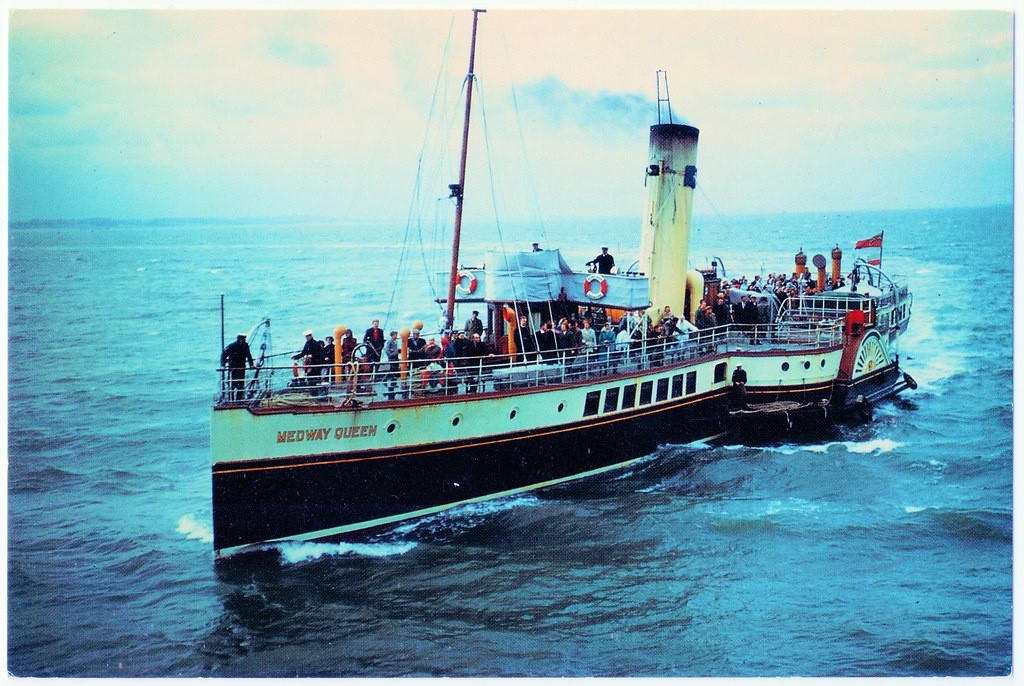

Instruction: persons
[220,333,255,400]
[532,243,541,251]
[695,267,860,356]
[585,247,615,275]
[291,305,700,394]
[731,364,747,410]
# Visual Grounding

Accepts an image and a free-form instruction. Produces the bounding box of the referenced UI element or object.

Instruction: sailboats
[211,10,920,560]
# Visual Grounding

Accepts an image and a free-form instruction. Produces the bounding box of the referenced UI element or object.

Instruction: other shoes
[391,385,399,388]
[382,381,388,386]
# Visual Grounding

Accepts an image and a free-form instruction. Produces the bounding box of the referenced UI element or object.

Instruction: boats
[491,364,567,386]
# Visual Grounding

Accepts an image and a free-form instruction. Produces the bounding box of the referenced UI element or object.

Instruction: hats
[303,330,312,337]
[412,329,420,334]
[736,362,742,368]
[236,333,246,339]
[705,306,713,310]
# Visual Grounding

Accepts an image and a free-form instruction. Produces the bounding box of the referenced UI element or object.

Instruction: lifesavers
[423,363,445,392]
[584,274,608,300]
[293,357,305,377]
[455,270,476,295]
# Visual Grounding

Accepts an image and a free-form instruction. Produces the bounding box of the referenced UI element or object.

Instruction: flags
[855,233,882,248]
[867,253,880,265]
